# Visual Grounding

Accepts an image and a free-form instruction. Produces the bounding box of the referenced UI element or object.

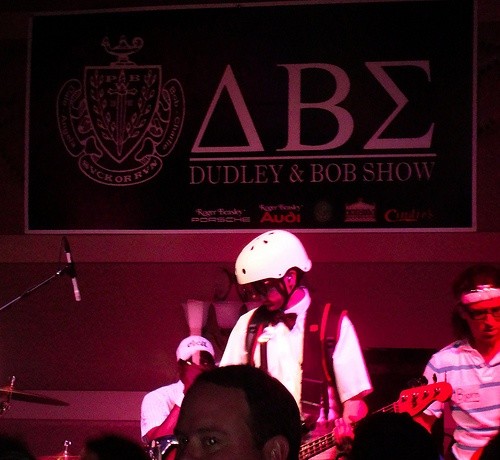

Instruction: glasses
[461,302,500,321]
[238,281,271,303]
[185,359,210,367]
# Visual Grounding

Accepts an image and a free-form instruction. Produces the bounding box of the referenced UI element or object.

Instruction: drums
[145,436,180,460]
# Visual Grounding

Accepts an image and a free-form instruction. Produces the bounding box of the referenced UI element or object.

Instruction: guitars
[298,371,454,460]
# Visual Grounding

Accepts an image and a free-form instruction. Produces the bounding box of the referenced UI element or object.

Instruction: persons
[78,433,153,460]
[0,431,35,460]
[218,229,373,460]
[139,334,215,460]
[408,262,500,460]
[479,431,500,460]
[173,364,301,460]
[344,411,441,460]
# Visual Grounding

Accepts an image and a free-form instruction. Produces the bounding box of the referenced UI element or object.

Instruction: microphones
[64,243,80,301]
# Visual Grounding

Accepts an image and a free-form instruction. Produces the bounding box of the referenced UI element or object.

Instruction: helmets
[234,227,313,286]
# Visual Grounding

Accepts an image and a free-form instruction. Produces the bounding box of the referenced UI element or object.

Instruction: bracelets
[175,393,185,407]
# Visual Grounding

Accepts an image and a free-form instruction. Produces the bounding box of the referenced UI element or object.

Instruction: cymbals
[0,387,71,406]
[35,450,83,460]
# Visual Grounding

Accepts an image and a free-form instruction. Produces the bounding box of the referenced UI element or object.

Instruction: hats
[176,335,215,362]
